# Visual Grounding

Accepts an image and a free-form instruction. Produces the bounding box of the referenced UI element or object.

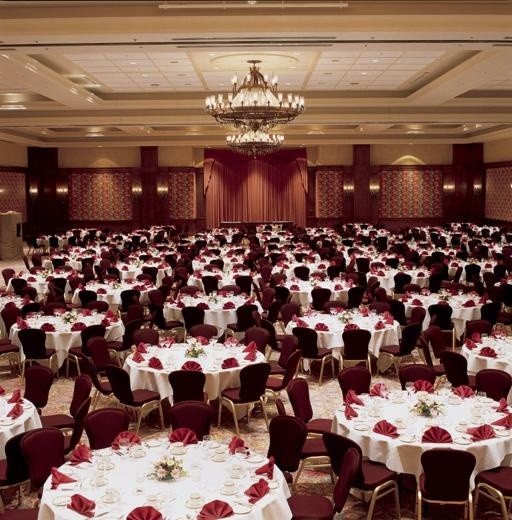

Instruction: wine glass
[302,306,386,326]
[479,326,512,350]
[142,335,246,361]
[180,290,246,307]
[26,309,99,326]
[410,287,479,305]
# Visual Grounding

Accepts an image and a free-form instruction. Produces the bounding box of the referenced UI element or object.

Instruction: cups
[85,278,146,289]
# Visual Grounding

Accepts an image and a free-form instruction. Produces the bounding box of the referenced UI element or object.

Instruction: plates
[51,432,280,516]
[0,420,14,426]
[352,381,511,445]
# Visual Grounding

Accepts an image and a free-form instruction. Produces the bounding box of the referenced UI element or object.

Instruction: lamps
[157,177,168,199]
[369,175,382,199]
[443,176,455,193]
[29,183,38,201]
[132,179,142,199]
[473,177,483,197]
[56,182,68,199]
[343,177,354,198]
[205,60,304,159]
